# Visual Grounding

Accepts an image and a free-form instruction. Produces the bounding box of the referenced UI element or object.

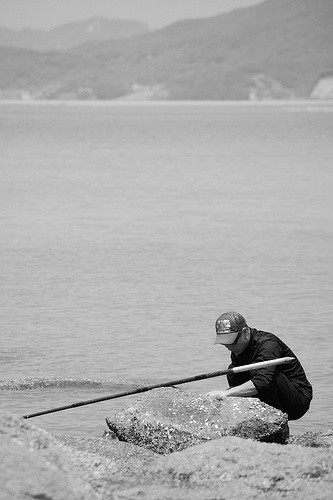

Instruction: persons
[204,311,313,421]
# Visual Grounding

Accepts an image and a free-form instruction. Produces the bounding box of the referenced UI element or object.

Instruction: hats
[215,311,247,344]
